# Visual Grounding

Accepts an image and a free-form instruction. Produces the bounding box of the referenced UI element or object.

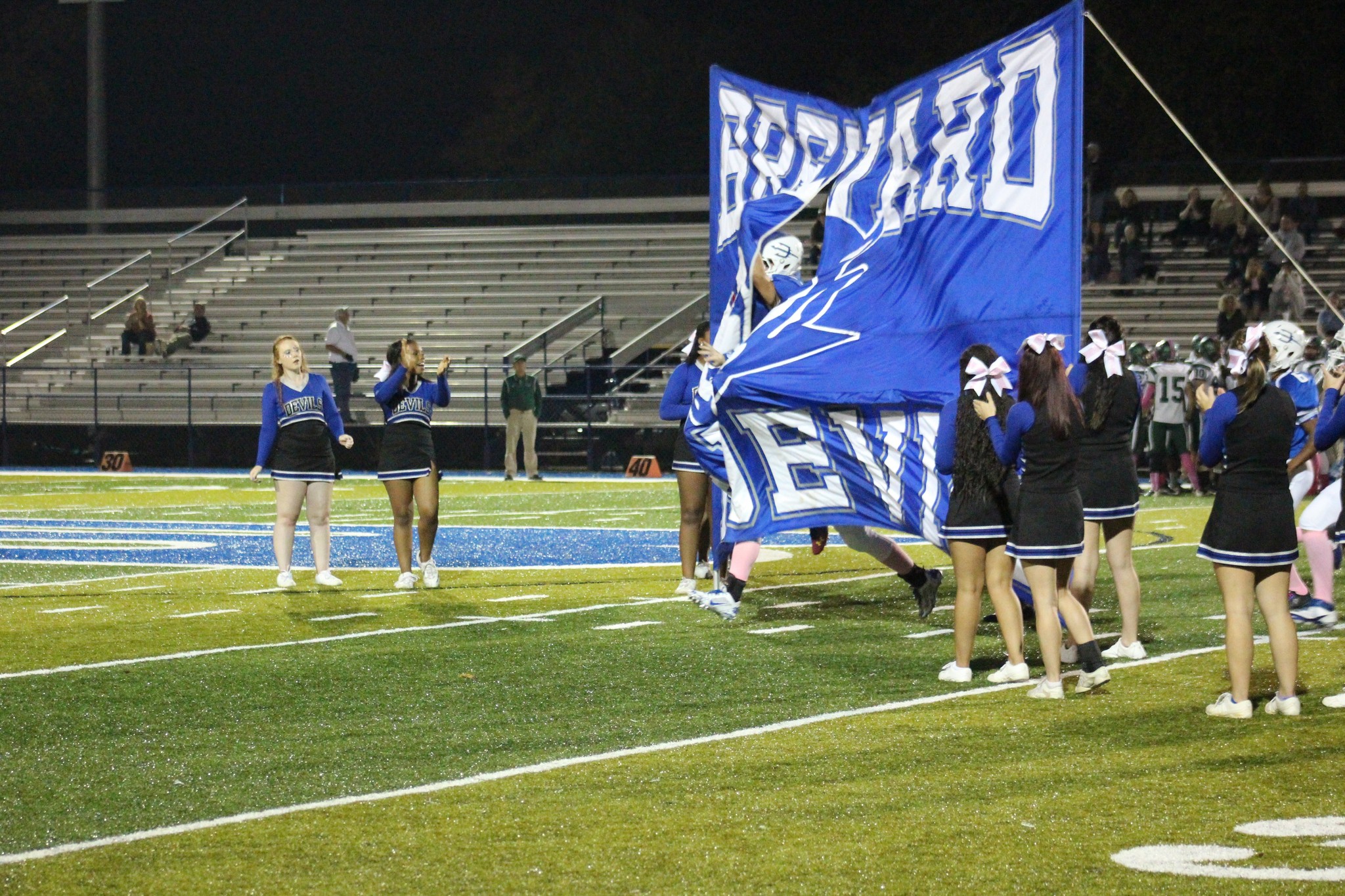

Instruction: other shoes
[505,474,513,481]
[981,606,1037,624]
[1138,478,1221,499]
[530,473,542,480]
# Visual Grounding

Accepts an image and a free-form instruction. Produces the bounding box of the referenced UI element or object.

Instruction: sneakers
[315,569,343,586]
[1290,598,1337,625]
[1322,692,1345,708]
[1100,638,1146,660]
[1059,643,1078,663]
[675,577,697,594]
[938,661,972,682]
[394,572,419,589]
[1075,666,1111,693]
[809,525,829,555]
[987,660,1029,683]
[416,550,440,588]
[688,585,727,609]
[1205,692,1253,719]
[1264,691,1300,716]
[701,592,741,620]
[1026,678,1065,699]
[1287,590,1313,610]
[277,566,296,588]
[694,560,713,579]
[908,568,943,619]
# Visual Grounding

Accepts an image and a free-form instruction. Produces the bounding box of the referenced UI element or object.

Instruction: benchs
[1080,224,1345,359]
[785,222,819,277]
[0,238,708,410]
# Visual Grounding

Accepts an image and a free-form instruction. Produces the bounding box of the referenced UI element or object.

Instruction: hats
[513,355,526,364]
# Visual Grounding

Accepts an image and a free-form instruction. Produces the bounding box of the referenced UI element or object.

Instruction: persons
[123,295,543,589]
[658,144,1345,718]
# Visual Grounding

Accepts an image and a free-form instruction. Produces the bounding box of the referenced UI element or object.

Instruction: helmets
[1264,319,1306,374]
[1129,333,1218,365]
[1302,336,1329,361]
